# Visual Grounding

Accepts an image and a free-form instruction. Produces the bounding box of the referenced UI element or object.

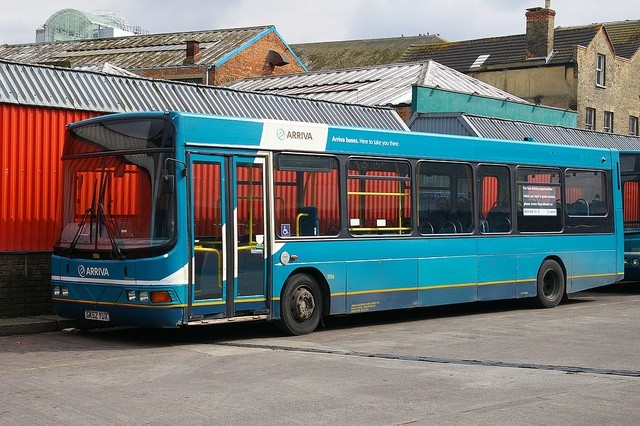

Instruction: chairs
[418,195,523,234]
[557,199,608,216]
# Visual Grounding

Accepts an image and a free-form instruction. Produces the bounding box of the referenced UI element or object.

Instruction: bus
[618,149,640,269]
[47,109,625,336]
